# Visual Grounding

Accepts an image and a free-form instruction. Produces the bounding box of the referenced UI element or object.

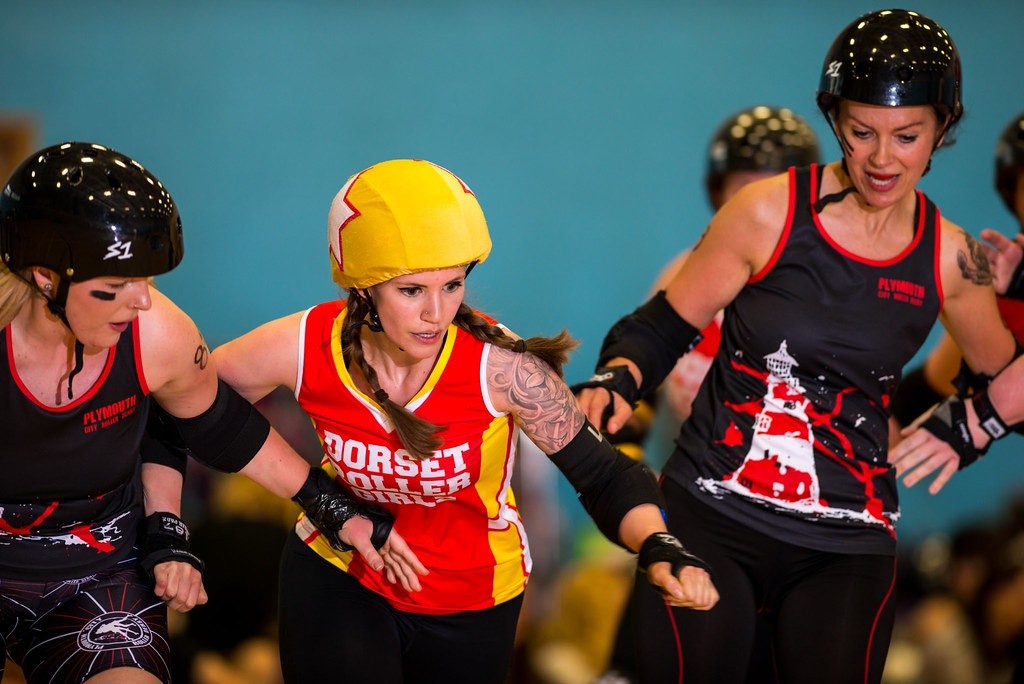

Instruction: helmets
[0,142,184,283]
[327,159,492,290]
[704,106,820,214]
[993,110,1024,217]
[816,9,963,124]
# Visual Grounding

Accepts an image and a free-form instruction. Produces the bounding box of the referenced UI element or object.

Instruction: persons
[1,139,430,684]
[569,7,1024,684]
[598,105,825,484]
[888,111,1024,496]
[137,158,718,684]
